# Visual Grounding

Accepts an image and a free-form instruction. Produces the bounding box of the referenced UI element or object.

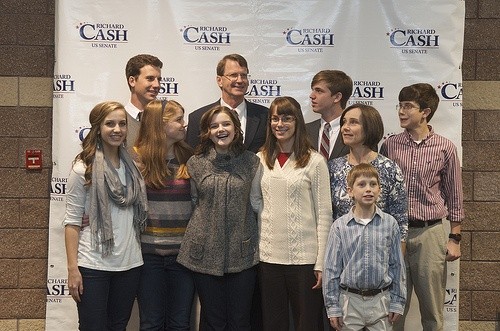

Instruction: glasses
[395,104,422,112]
[219,72,252,83]
[161,99,168,113]
[266,114,296,125]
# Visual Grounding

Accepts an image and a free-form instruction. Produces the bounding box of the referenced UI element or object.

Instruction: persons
[82,54,409,331]
[379,83,465,331]
[64,101,143,331]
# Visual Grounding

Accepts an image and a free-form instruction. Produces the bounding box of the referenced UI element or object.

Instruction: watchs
[448,233,461,241]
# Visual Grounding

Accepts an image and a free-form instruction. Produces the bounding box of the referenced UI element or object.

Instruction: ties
[233,110,239,118]
[319,122,331,161]
[138,112,142,121]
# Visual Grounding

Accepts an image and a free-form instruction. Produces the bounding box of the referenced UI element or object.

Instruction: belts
[339,284,389,296]
[408,219,443,228]
